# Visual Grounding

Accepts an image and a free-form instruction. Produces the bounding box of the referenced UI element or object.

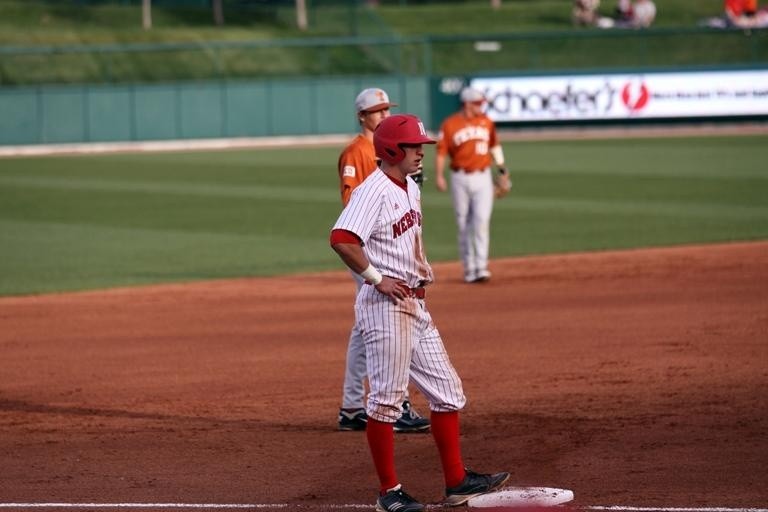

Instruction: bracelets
[359,263,383,289]
[498,166,507,176]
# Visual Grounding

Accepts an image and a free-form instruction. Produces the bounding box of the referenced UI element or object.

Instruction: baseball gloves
[493,174,513,199]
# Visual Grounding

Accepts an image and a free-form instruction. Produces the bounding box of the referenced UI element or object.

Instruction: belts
[448,163,486,175]
[362,277,426,300]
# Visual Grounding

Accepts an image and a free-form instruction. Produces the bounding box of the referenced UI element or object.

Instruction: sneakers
[336,402,370,433]
[390,399,433,434]
[375,486,427,512]
[443,464,510,506]
[461,266,492,284]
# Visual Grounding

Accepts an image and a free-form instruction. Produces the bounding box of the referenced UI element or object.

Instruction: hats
[353,87,399,114]
[457,86,488,105]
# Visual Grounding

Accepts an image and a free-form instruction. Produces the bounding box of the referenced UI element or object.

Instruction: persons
[723,0,758,28]
[431,86,511,284]
[330,113,512,512]
[614,0,657,29]
[337,84,431,436]
[569,0,611,29]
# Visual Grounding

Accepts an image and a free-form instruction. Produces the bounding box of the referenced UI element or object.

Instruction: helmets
[370,112,437,166]
